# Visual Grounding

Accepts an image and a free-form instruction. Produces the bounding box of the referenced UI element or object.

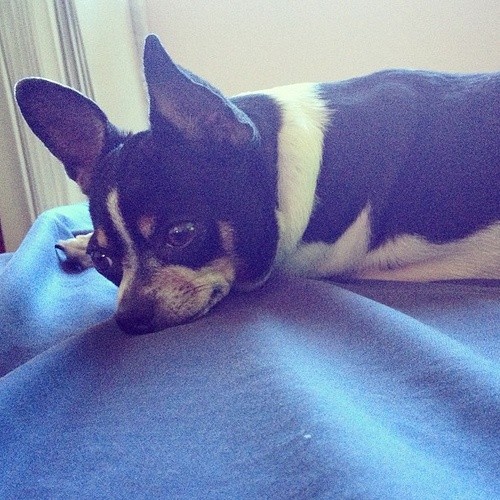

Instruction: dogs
[14,33,500,336]
